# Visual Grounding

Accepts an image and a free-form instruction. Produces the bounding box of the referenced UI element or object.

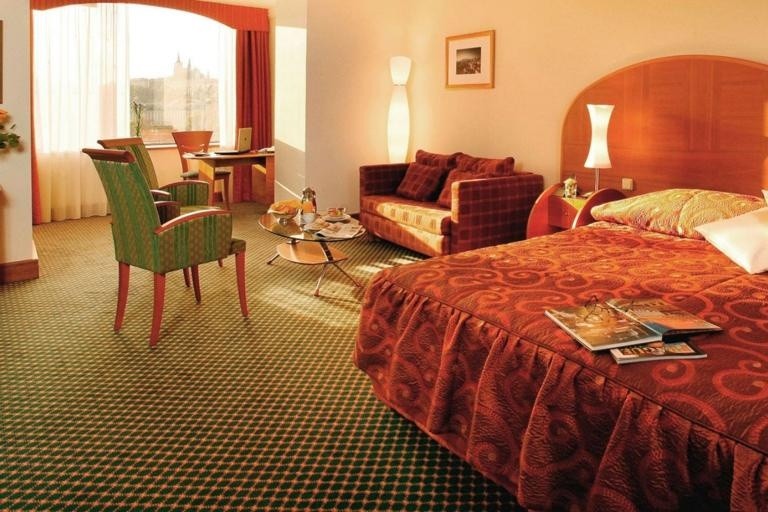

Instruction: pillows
[692,205,768,280]
[396,145,517,212]
[587,181,768,250]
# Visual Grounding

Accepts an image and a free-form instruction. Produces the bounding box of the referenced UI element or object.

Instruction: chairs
[170,130,233,212]
[96,138,226,270]
[80,147,250,348]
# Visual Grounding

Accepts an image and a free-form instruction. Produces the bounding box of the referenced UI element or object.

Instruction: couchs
[359,151,546,257]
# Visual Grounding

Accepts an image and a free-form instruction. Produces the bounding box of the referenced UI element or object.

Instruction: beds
[359,54,768,511]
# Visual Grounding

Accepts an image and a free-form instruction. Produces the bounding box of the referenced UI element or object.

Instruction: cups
[303,212,315,223]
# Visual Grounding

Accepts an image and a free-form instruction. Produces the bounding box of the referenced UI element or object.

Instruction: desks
[183,150,276,205]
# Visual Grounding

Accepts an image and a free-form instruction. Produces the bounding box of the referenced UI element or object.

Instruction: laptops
[214,128,252,154]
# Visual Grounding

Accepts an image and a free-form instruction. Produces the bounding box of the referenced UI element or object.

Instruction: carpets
[1,206,526,506]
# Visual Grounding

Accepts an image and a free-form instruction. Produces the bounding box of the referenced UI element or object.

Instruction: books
[544,298,722,364]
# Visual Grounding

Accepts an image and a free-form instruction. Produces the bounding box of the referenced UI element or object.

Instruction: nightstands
[524,181,626,241]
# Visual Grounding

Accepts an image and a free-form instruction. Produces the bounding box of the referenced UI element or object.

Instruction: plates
[323,212,352,221]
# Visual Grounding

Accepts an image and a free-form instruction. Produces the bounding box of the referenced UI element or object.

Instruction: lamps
[583,105,614,199]
[385,53,415,166]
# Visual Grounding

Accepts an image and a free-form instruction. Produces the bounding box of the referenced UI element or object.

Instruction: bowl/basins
[269,208,299,221]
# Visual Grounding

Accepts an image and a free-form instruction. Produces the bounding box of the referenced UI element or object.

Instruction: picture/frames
[444,29,496,91]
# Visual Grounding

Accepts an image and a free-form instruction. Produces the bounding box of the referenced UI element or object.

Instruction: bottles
[562,177,578,198]
[299,187,318,215]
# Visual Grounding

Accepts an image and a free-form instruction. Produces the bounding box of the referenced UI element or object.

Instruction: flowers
[0,110,23,152]
